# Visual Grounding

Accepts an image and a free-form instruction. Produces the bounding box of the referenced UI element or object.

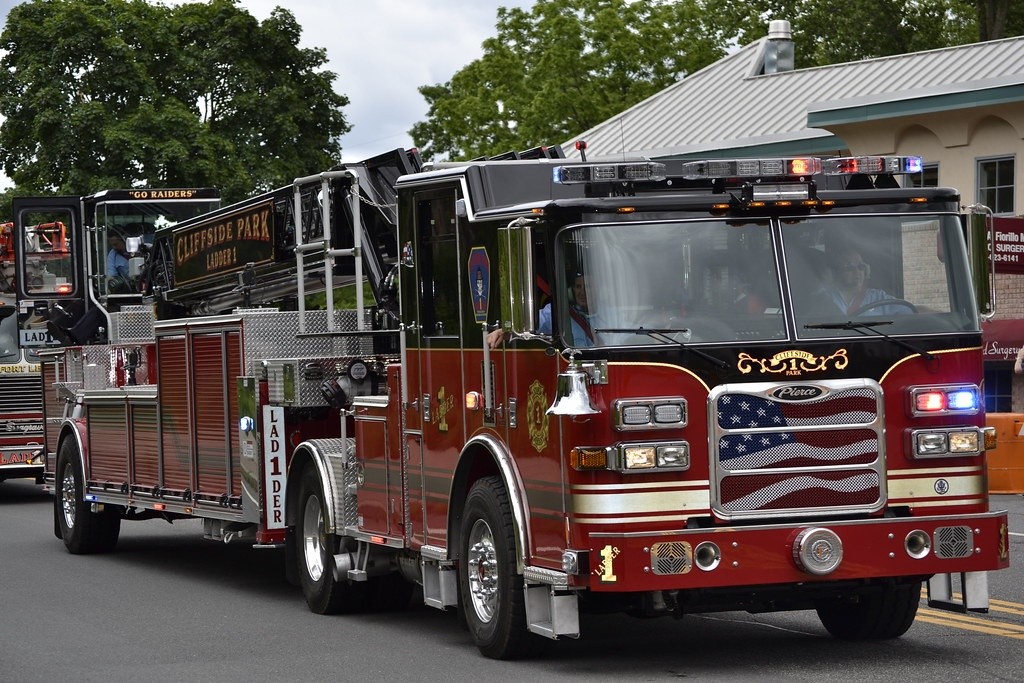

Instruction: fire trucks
[0,137,1015,664]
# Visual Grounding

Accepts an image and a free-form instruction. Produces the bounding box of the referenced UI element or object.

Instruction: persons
[106,228,142,303]
[801,245,916,317]
[485,261,624,349]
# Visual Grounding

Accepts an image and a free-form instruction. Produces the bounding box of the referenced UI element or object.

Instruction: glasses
[842,263,867,271]
[577,272,584,276]
